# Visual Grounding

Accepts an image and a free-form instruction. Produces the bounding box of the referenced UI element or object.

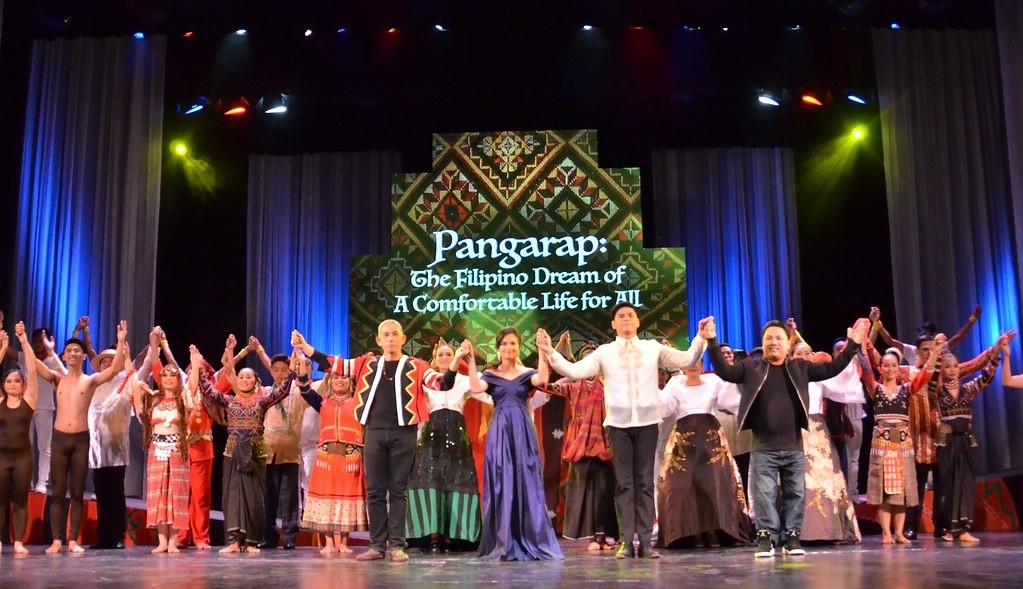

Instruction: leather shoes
[442,543,451,552]
[89,542,112,549]
[112,540,124,548]
[283,539,294,549]
[256,537,277,549]
[428,544,440,553]
[904,529,916,539]
[933,527,959,537]
[615,542,635,559]
[637,544,659,558]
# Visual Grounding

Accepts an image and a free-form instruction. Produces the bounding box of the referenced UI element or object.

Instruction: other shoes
[390,550,409,561]
[355,550,385,560]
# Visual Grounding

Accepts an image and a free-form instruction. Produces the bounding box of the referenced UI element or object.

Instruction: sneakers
[781,528,805,554]
[753,530,775,557]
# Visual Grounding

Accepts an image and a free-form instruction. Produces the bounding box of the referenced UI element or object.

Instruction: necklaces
[383,361,398,381]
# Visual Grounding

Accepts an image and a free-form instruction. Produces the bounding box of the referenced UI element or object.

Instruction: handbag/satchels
[233,442,268,479]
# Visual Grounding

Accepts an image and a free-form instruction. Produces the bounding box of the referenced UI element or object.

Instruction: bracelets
[878,320,882,329]
[968,314,976,322]
[871,320,879,330]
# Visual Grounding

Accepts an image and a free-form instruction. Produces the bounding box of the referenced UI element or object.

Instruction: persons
[702,318,866,560]
[291,319,471,560]
[535,302,714,559]
[653,333,764,544]
[787,306,1023,543]
[0,309,367,556]
[405,337,620,553]
[462,327,565,562]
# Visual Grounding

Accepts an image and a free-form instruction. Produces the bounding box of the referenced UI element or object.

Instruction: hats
[90,349,116,372]
